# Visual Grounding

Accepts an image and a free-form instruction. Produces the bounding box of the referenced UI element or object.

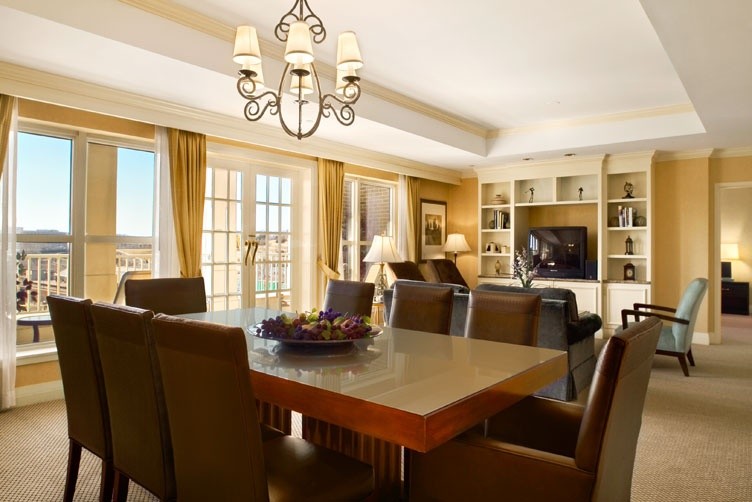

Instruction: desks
[14,313,53,343]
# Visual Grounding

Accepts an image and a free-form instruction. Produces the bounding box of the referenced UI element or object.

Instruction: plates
[246,320,384,345]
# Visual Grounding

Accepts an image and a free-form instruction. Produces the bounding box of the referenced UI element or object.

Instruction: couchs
[381,279,603,401]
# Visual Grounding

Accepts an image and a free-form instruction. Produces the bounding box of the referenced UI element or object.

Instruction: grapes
[256,308,372,340]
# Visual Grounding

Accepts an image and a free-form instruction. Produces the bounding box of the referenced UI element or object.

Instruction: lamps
[362,234,404,297]
[235,1,363,139]
[442,233,473,265]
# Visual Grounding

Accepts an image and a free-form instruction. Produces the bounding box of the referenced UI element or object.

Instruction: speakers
[585,260,596,280]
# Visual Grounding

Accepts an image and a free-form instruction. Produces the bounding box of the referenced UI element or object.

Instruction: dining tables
[157,301,576,500]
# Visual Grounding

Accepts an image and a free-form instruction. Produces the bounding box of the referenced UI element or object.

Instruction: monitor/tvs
[527,226,585,278]
[721,262,732,277]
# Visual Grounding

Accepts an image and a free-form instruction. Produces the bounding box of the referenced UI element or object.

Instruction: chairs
[45,292,115,501]
[385,281,457,338]
[424,257,471,290]
[614,275,708,377]
[463,287,543,349]
[406,316,668,501]
[323,277,378,323]
[152,310,381,502]
[89,301,286,502]
[387,260,428,284]
[123,274,208,318]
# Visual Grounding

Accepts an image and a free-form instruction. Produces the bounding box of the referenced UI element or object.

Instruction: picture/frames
[418,198,462,264]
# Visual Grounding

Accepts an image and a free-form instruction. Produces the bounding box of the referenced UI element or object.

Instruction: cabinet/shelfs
[721,280,750,315]
[476,151,657,339]
